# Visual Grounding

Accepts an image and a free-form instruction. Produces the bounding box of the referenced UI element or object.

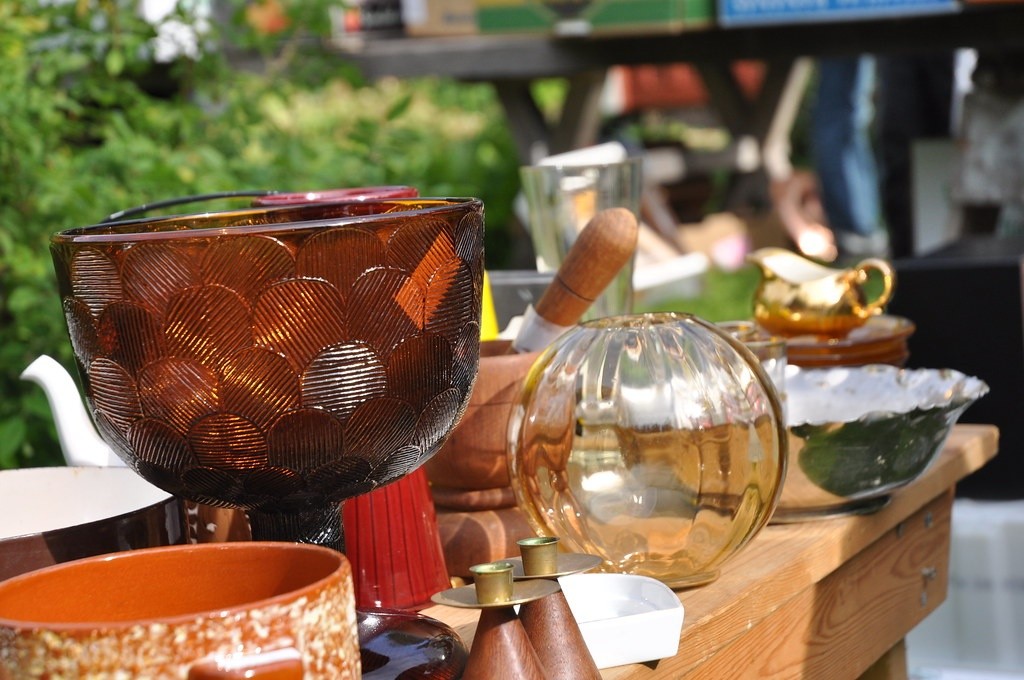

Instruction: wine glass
[48,197,486,679]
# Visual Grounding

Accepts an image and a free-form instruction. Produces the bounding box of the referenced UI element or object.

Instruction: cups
[468,563,514,604]
[521,158,642,321]
[517,538,560,575]
[714,320,787,418]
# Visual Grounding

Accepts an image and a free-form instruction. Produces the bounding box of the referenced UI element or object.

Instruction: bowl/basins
[0,468,191,581]
[766,363,989,522]
[0,543,363,680]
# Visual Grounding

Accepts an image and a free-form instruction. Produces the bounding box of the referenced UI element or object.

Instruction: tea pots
[15,353,132,468]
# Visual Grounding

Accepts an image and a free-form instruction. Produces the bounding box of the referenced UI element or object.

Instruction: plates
[783,315,915,369]
[500,554,604,579]
[430,579,564,605]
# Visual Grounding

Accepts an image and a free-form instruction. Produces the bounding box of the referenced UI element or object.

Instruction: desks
[342,0,1022,270]
[420,421,1002,680]
